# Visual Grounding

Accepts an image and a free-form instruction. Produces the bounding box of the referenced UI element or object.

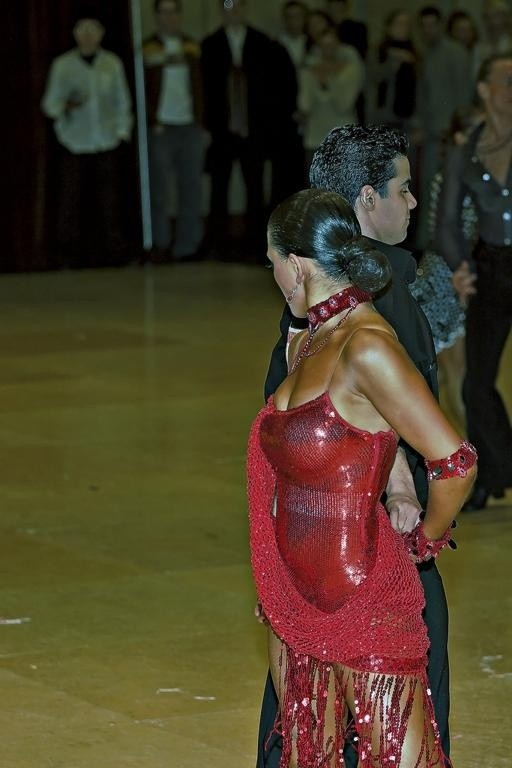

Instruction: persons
[254,123,452,768]
[434,49,512,511]
[242,187,481,768]
[407,95,488,430]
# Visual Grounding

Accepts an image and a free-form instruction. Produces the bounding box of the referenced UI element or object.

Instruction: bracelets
[404,511,457,564]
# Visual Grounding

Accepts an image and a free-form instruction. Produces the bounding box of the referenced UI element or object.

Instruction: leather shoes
[459,485,504,510]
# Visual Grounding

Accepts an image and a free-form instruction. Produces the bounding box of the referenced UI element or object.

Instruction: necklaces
[283,284,376,375]
